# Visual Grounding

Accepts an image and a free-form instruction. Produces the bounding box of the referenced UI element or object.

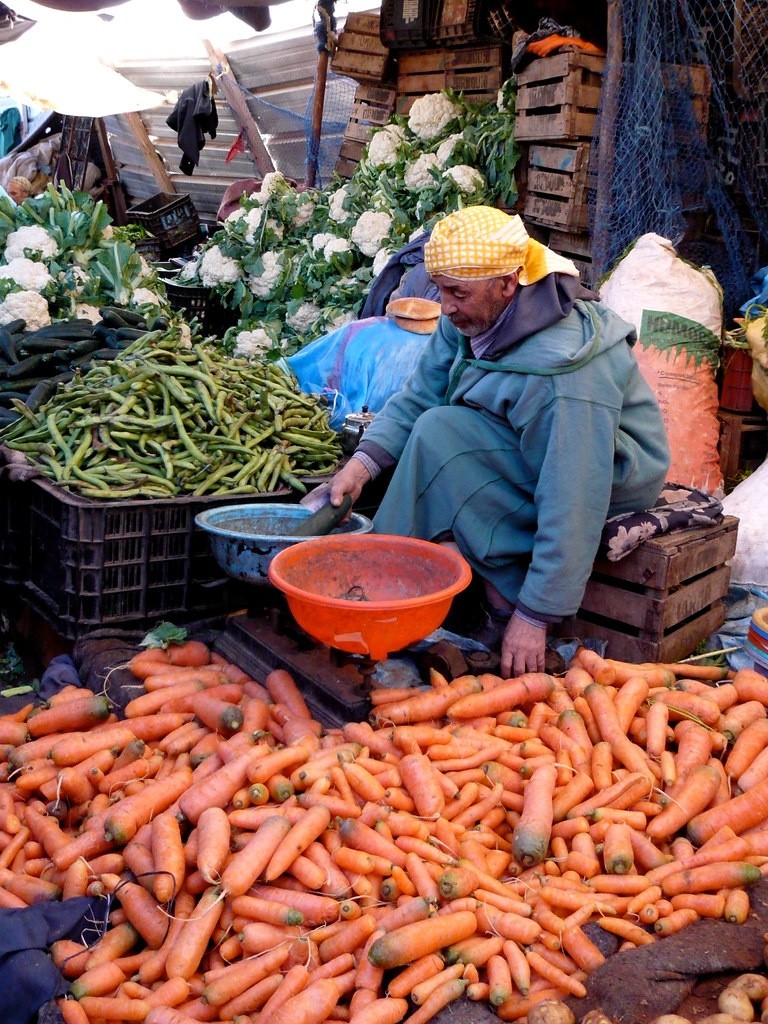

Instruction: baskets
[158,269,240,332]
[125,190,204,262]
[0,448,344,667]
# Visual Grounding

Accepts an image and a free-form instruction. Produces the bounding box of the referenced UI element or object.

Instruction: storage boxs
[330,0,712,286]
[575,515,739,665]
[718,347,755,413]
[125,192,240,339]
[0,477,293,639]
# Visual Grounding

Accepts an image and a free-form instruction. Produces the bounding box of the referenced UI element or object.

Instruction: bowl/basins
[194,504,374,585]
[744,606,768,679]
[268,534,473,661]
[386,298,442,335]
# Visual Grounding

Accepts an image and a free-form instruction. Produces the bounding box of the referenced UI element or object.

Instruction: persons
[328,205,670,678]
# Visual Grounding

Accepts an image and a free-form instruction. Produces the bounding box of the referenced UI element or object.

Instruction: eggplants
[0,307,170,428]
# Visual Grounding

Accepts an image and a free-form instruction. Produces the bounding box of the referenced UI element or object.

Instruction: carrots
[0,639,767,1024]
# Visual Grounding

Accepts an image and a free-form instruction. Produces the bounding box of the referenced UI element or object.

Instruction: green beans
[0,328,344,500]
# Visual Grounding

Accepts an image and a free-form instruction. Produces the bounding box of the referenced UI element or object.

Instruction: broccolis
[0,87,520,361]
